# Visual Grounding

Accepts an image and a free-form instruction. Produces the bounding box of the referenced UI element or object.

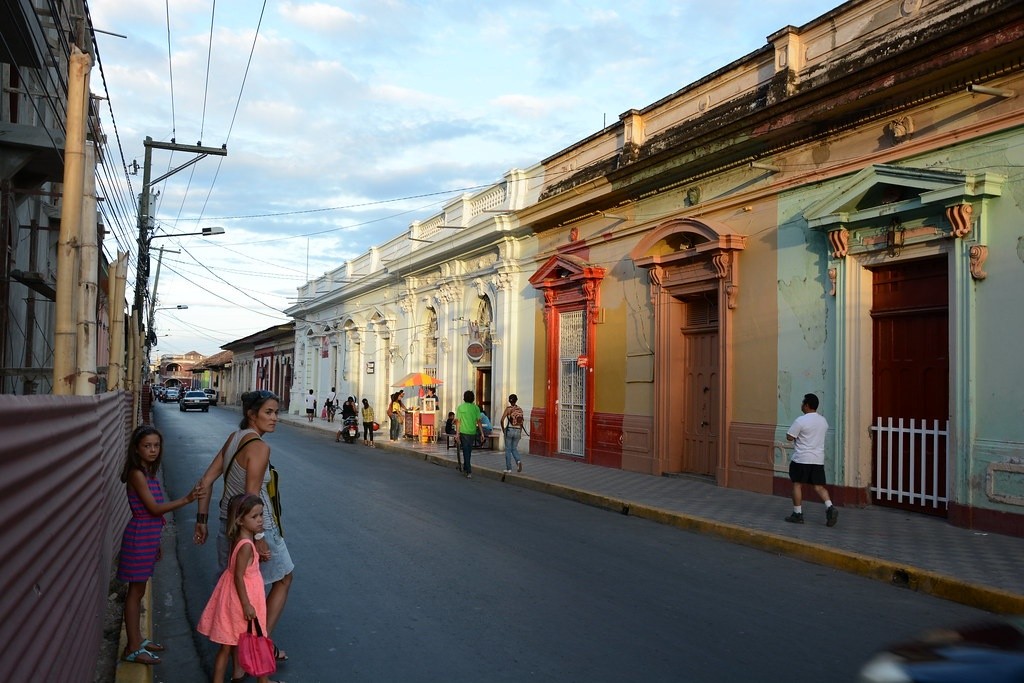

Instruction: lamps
[885,218,905,258]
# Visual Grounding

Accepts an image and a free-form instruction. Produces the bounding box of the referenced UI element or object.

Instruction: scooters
[334,405,361,444]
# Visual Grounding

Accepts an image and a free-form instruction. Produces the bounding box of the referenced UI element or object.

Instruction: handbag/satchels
[238,616,276,676]
[267,467,282,537]
[327,400,333,408]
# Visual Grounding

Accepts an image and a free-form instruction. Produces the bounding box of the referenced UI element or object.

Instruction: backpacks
[506,404,524,426]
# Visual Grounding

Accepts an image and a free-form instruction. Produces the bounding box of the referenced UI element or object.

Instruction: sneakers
[826,507,839,527]
[785,511,804,523]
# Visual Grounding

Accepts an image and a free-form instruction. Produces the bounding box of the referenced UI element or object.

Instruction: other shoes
[370,444,376,449]
[517,460,523,473]
[336,439,339,442]
[466,473,471,478]
[503,469,511,473]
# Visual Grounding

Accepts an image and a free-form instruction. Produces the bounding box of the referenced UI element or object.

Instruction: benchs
[442,432,499,450]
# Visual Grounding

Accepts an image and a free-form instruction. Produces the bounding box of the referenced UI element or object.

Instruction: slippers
[138,636,163,652]
[231,667,248,681]
[273,645,290,661]
[262,679,285,683]
[121,647,161,664]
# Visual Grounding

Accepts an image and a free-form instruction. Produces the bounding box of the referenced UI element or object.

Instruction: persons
[501,394,524,473]
[120,425,206,665]
[326,387,337,423]
[455,391,485,478]
[427,388,439,410]
[361,399,374,447]
[785,394,839,527]
[445,412,457,447]
[476,405,492,448]
[193,391,295,683]
[336,397,358,442]
[196,494,286,683]
[306,389,315,421]
[387,390,407,440]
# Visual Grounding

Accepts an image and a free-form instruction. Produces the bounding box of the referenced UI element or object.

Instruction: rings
[197,537,200,539]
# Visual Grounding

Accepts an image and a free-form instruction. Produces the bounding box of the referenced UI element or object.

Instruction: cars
[152,383,218,407]
[179,390,210,412]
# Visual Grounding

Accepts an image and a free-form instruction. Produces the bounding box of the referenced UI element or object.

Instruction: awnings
[390,371,444,410]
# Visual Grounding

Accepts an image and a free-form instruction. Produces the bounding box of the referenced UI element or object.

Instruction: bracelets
[197,512,208,524]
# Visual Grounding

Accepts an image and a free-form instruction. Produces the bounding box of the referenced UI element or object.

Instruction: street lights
[131,226,226,334]
[141,305,189,392]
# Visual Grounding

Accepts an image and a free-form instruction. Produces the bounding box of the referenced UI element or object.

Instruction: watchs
[254,532,264,540]
